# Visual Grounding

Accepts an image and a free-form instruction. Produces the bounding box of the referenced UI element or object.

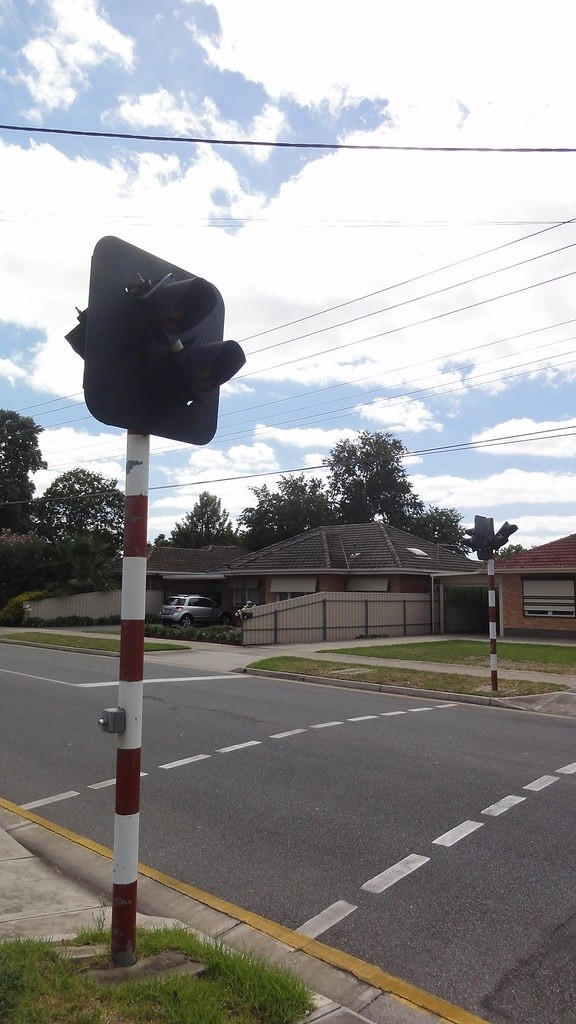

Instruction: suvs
[158,592,232,628]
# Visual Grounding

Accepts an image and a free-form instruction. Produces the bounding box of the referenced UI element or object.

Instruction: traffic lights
[462,514,488,560]
[83,235,246,446]
[487,521,518,551]
